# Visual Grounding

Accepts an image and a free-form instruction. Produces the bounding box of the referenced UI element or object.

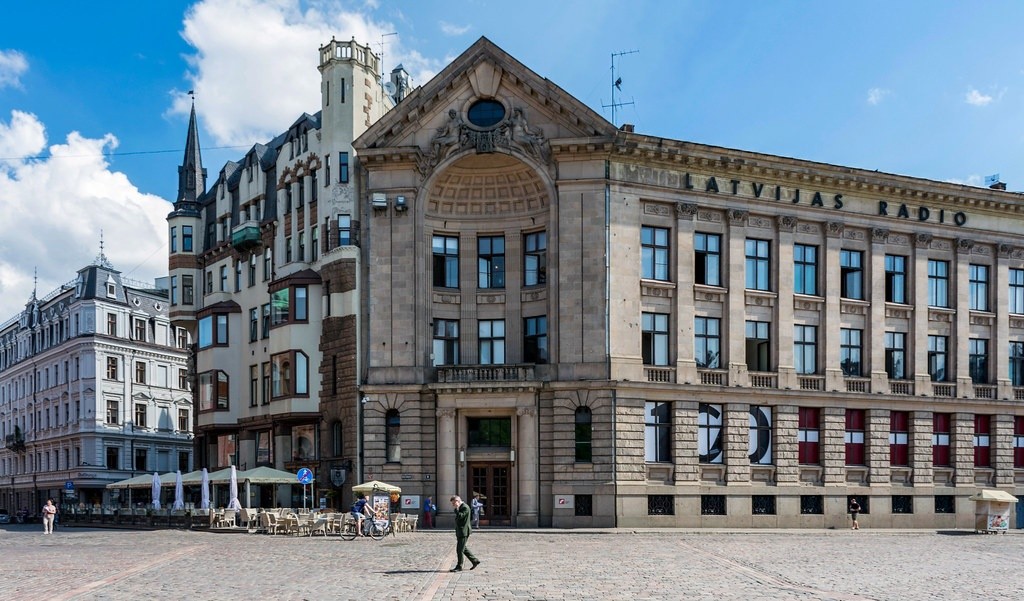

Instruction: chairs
[398,513,405,533]
[407,514,418,532]
[218,507,365,537]
[391,513,400,534]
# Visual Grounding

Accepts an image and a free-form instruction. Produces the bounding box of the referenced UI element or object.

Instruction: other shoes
[450,566,462,572]
[43,532,48,534]
[49,532,52,534]
[359,533,365,537]
[470,561,480,570]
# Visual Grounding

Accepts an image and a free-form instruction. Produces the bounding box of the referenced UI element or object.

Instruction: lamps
[510,446,515,466]
[460,447,465,467]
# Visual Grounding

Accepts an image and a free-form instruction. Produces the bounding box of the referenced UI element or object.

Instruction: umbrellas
[151,472,161,510]
[351,480,401,515]
[173,469,185,510]
[473,491,487,500]
[200,468,210,509]
[228,465,242,515]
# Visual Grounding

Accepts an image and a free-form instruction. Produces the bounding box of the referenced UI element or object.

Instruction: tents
[105,466,315,509]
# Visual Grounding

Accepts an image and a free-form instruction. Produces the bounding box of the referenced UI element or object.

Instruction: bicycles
[339,512,386,540]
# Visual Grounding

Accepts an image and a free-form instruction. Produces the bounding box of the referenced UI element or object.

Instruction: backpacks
[350,500,366,507]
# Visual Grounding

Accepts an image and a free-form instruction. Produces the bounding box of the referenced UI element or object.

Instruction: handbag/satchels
[471,509,474,514]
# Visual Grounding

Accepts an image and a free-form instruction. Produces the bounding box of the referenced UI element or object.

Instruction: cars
[0,508,10,524]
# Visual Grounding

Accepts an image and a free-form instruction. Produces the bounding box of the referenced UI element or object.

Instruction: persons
[423,108,463,165]
[449,495,480,572]
[470,494,483,529]
[15,498,100,534]
[504,106,549,167]
[849,499,861,530]
[352,495,378,536]
[423,495,434,529]
